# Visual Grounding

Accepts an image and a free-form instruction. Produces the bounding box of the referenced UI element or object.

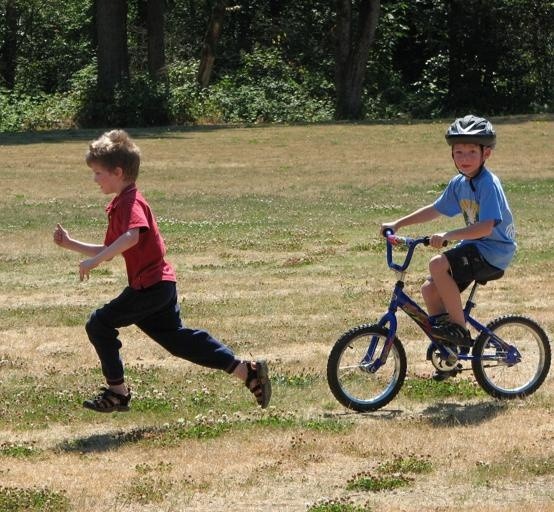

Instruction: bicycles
[328,228,551,413]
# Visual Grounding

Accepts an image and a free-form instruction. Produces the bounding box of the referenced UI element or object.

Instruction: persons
[380,114,517,381]
[51,129,270,414]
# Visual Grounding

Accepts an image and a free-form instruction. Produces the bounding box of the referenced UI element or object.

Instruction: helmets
[445,114,497,146]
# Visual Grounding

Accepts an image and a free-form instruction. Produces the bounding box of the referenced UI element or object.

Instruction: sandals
[83,387,132,413]
[244,360,273,409]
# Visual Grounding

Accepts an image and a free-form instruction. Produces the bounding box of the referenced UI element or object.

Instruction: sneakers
[430,320,471,347]
[433,363,463,381]
